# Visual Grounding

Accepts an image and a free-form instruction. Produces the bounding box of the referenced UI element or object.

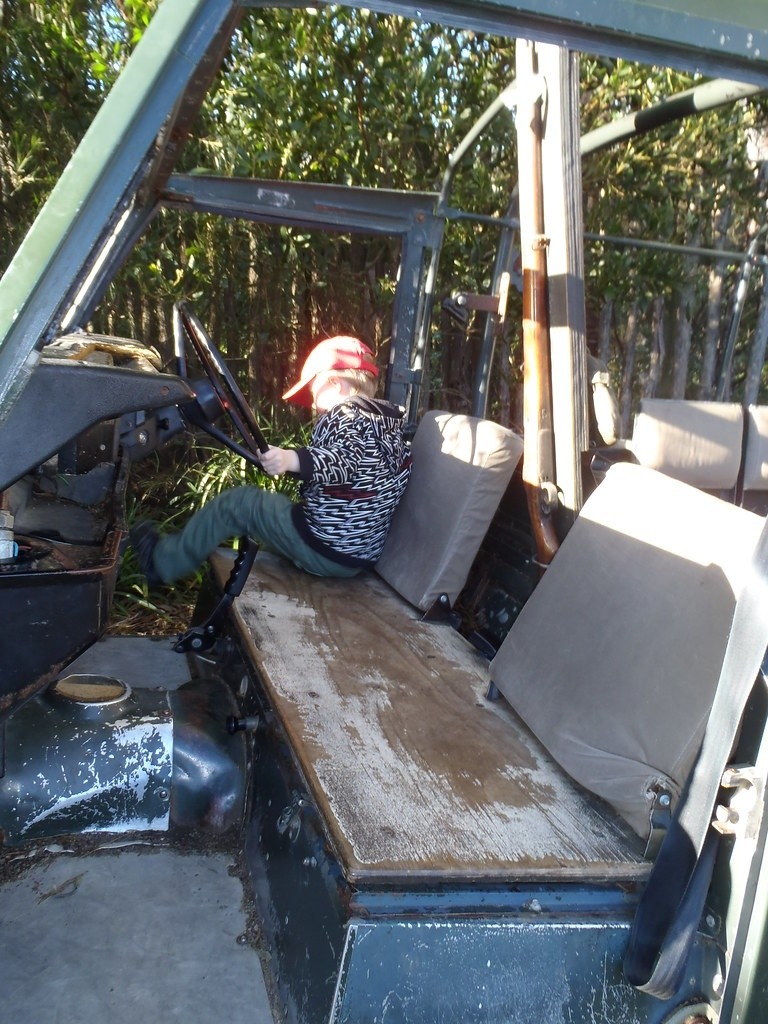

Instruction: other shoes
[131,525,163,585]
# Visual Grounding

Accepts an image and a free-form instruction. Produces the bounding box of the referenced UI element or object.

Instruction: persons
[130,335,413,588]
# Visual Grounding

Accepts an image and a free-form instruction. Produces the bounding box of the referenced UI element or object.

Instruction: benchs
[579,401,768,516]
[168,399,768,1024]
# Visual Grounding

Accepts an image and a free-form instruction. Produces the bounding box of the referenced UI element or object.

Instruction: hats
[282,335,379,408]
